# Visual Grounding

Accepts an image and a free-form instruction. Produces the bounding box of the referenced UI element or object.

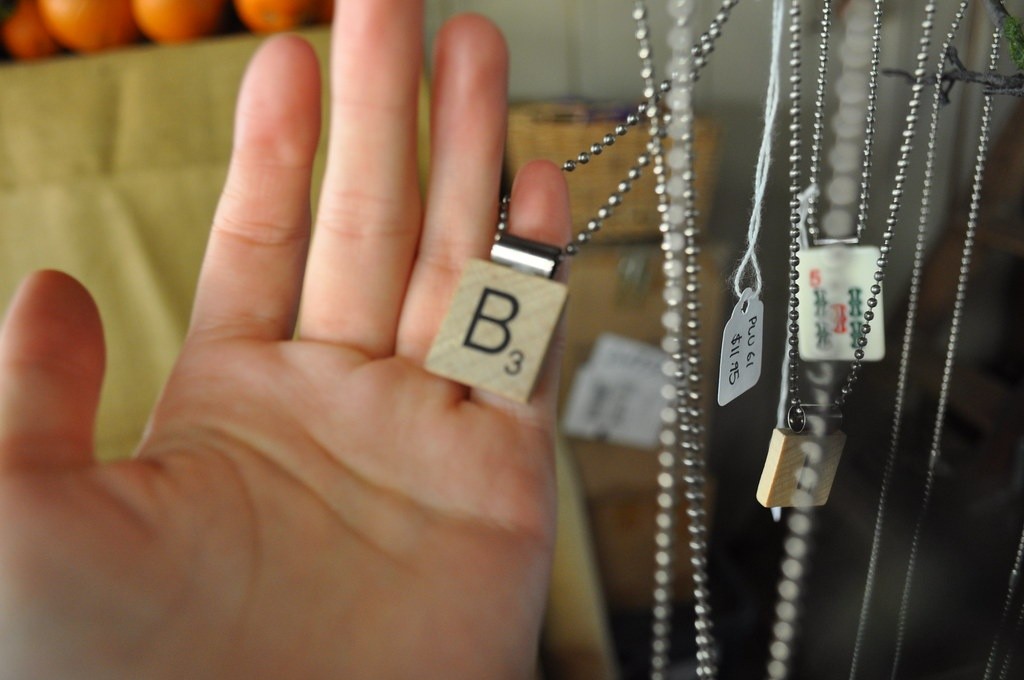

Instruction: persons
[0,0,570,680]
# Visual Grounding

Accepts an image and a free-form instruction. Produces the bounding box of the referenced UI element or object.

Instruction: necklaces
[424,0,1024,680]
